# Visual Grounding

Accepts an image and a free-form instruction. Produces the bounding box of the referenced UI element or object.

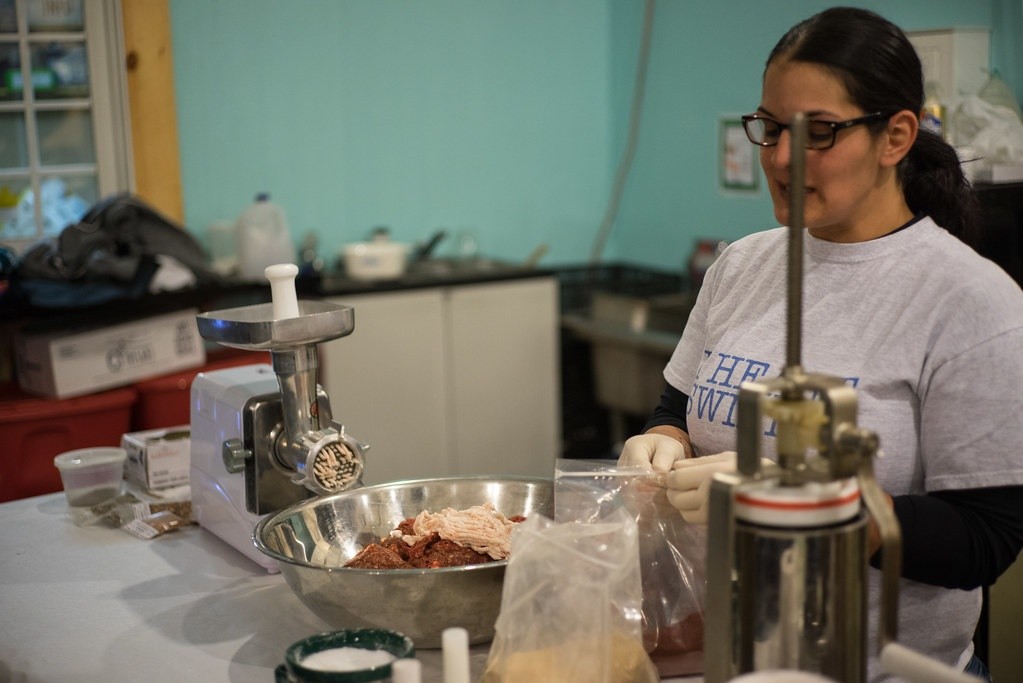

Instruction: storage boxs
[121,424,192,490]
[14,308,207,399]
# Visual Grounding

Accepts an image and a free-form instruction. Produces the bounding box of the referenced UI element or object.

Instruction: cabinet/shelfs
[323,275,562,490]
[905,25,993,146]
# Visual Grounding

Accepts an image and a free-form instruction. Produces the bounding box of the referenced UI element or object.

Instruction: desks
[0,482,492,683]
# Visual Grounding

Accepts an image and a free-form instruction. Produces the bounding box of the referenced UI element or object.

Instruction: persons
[615,6,1023,683]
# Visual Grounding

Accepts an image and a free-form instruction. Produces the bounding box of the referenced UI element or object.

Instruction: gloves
[617,430,685,496]
[660,450,777,524]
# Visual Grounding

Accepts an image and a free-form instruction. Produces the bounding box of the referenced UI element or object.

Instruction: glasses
[741,111,886,150]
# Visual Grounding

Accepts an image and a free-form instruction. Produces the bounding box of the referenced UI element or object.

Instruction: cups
[208,223,239,274]
[274,626,415,683]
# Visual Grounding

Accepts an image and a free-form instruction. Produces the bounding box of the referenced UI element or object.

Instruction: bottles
[240,193,296,281]
[297,230,325,289]
[976,66,1022,115]
[919,80,947,140]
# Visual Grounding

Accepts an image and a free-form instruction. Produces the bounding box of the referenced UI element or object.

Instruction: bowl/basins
[54,447,127,506]
[252,475,554,649]
[339,241,413,282]
